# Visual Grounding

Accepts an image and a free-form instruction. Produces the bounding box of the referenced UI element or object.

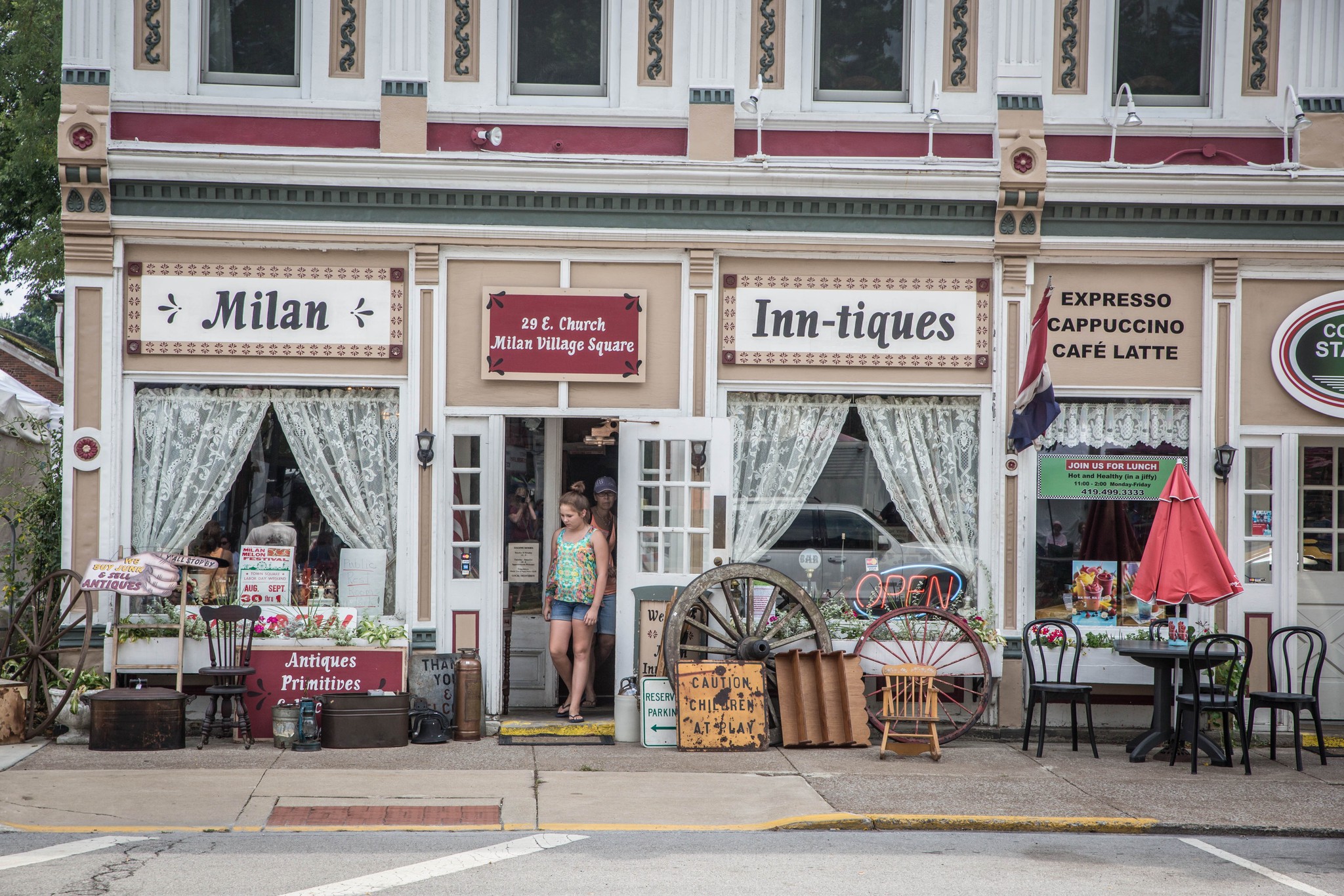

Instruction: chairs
[197,605,262,749]
[1149,618,1235,757]
[1022,618,1099,758]
[880,663,942,761]
[1240,626,1327,771]
[1170,633,1252,775]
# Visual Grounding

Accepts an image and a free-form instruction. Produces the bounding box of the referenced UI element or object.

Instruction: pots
[80,678,197,751]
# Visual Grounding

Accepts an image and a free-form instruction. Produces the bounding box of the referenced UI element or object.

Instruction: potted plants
[49,665,111,745]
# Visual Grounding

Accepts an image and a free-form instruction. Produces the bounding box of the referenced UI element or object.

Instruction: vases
[105,620,412,673]
[763,638,1003,677]
[1028,631,1214,684]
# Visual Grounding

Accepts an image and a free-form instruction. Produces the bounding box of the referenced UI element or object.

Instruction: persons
[198,533,240,605]
[310,531,340,604]
[507,476,543,614]
[211,522,240,547]
[244,496,298,581]
[877,500,918,541]
[1263,524,1271,535]
[1074,521,1087,553]
[544,478,618,724]
[1046,520,1068,547]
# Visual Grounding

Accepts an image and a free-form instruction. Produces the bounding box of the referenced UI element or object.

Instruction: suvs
[734,496,976,621]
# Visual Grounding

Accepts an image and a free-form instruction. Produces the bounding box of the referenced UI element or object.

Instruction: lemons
[1073,586,1078,593]
[1103,595,1111,603]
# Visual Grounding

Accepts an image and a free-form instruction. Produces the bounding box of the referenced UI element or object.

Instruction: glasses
[220,540,230,546]
[596,491,615,497]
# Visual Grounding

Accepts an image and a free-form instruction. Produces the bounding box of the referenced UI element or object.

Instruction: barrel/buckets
[271,702,301,748]
[614,695,640,742]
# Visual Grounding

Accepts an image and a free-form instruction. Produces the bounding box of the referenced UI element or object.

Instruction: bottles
[292,564,336,606]
[617,676,640,696]
[730,580,744,617]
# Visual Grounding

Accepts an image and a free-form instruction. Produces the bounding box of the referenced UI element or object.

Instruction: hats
[594,476,617,494]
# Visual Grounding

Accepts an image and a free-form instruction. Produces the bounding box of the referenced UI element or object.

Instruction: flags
[1011,286,1060,450]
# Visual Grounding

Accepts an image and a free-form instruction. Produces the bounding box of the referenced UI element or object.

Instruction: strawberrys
[1096,567,1102,570]
[1085,586,1092,592]
[1107,574,1112,578]
[1086,599,1117,618]
[1110,594,1115,598]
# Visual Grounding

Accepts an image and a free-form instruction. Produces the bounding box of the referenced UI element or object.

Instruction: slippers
[581,698,597,708]
[556,700,570,717]
[568,709,584,723]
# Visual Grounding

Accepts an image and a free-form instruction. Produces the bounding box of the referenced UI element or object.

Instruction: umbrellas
[1078,500,1143,561]
[1126,458,1246,733]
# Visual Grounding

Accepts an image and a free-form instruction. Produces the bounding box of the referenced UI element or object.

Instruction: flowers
[98,576,408,648]
[728,558,1008,652]
[1020,620,1247,689]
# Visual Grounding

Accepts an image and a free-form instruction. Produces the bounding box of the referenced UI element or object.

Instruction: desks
[1114,639,1247,767]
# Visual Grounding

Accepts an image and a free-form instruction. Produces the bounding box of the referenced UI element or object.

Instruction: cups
[1124,591,1139,614]
[1083,586,1103,611]
[1075,577,1094,599]
[1087,567,1104,584]
[1152,605,1159,613]
[1136,598,1153,620]
[1096,574,1115,598]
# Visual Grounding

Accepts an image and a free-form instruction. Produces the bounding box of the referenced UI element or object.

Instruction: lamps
[741,73,1314,168]
[471,126,503,146]
[691,441,707,473]
[415,428,436,470]
[1213,442,1238,484]
